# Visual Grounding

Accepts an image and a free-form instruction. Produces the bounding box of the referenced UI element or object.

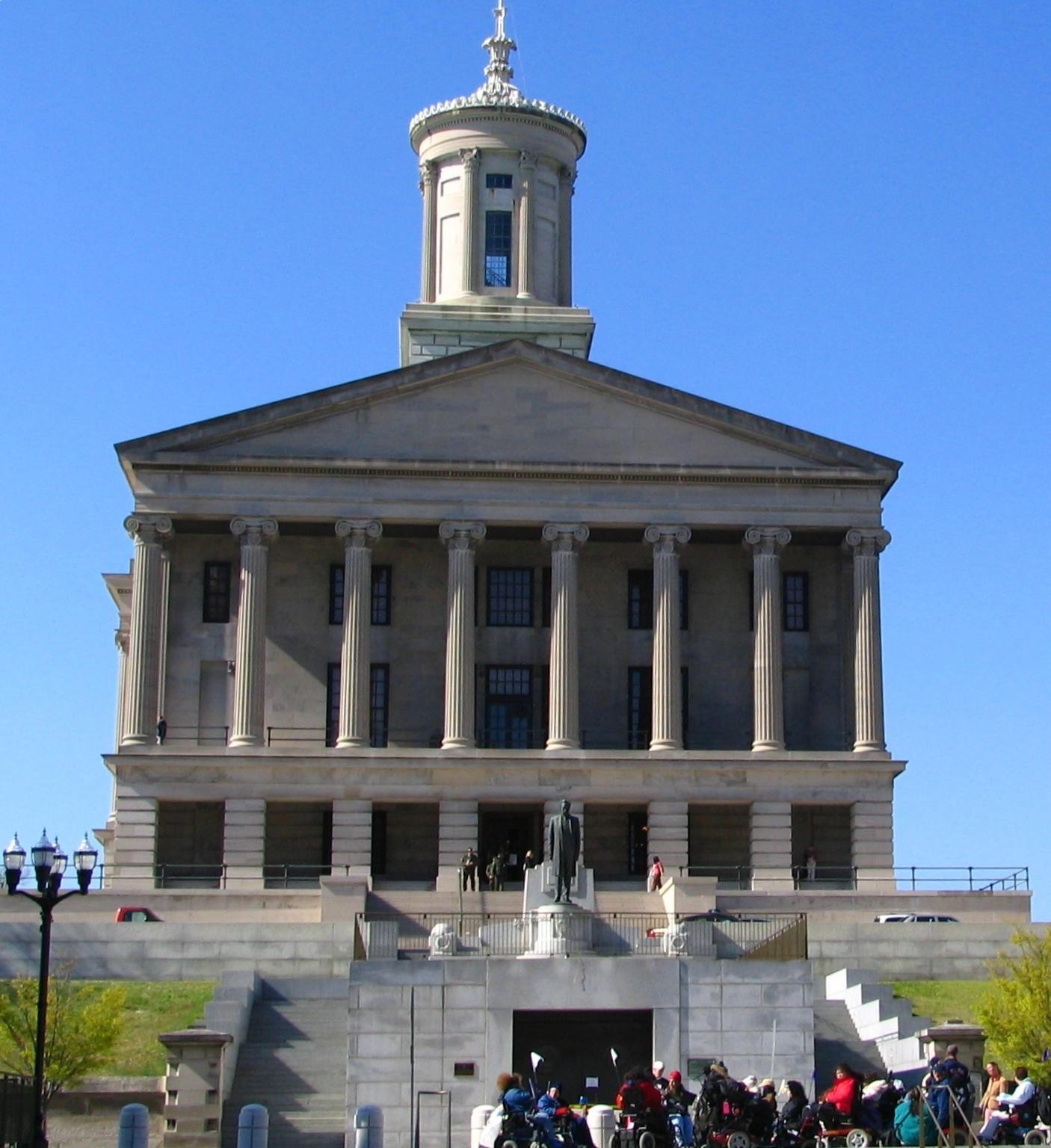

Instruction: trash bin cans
[0,1070,38,1146]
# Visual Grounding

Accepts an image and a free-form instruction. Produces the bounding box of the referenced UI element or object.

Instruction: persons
[486,854,507,892]
[616,1044,976,1148]
[461,849,478,892]
[979,1062,1008,1124]
[548,800,580,904]
[650,856,664,892]
[495,1072,598,1148]
[523,851,534,875]
[804,846,818,879]
[973,1066,1036,1145]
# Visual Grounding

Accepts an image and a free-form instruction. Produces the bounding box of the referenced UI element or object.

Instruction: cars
[681,914,772,922]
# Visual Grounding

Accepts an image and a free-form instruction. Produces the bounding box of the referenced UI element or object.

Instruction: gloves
[556,1106,570,1115]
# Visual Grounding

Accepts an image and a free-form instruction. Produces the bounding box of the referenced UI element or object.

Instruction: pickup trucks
[116,906,165,923]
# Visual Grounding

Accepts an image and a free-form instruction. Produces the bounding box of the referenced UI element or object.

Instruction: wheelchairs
[494,1099,586,1148]
[693,1079,878,1148]
[975,1100,1047,1147]
[608,1094,693,1148]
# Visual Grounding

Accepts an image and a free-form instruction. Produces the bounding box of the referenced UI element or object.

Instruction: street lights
[3,825,99,1148]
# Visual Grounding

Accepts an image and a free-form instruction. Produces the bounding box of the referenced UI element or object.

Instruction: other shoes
[973,1136,990,1146]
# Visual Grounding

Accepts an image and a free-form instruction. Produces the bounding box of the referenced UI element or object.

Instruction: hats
[670,1071,682,1082]
[550,1082,562,1093]
[931,1063,946,1073]
[759,1079,775,1088]
[741,1074,757,1089]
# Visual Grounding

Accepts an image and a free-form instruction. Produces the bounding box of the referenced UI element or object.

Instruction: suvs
[873,912,960,923]
